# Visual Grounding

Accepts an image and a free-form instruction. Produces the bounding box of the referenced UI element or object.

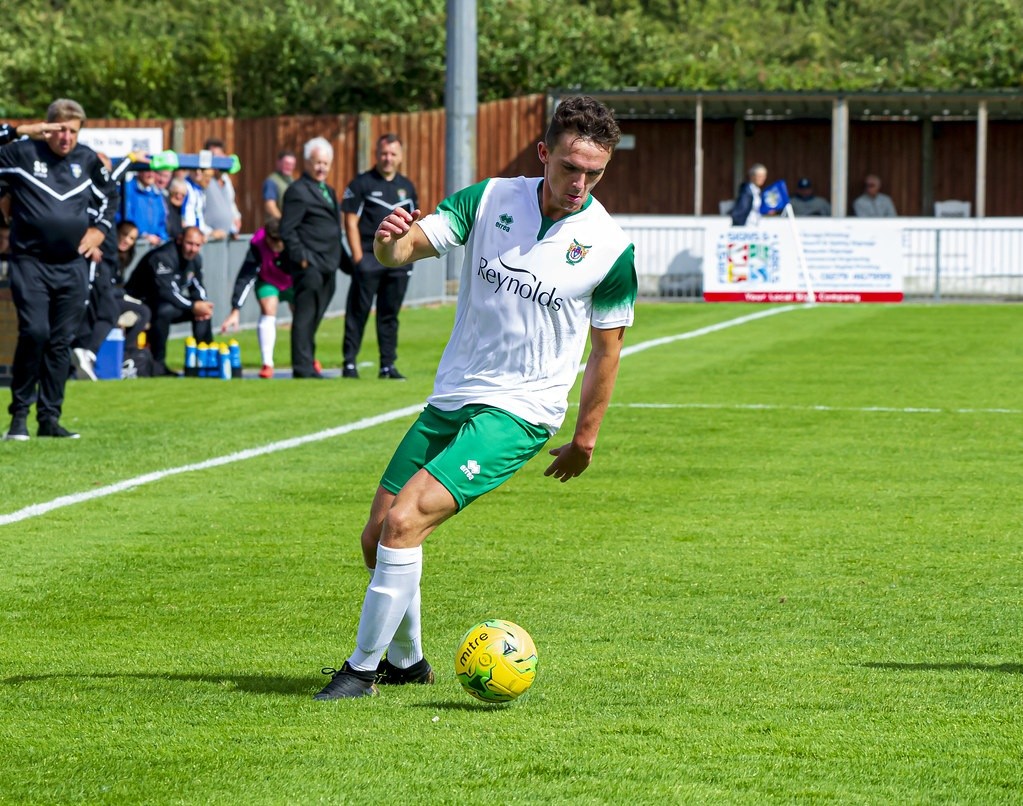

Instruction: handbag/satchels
[124,348,154,377]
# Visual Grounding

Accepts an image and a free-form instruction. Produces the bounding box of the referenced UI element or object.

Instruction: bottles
[184,336,242,380]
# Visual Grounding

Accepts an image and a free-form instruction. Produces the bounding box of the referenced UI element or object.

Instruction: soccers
[454,618,540,703]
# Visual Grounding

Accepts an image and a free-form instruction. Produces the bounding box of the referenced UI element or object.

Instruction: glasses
[268,234,281,243]
[865,183,876,188]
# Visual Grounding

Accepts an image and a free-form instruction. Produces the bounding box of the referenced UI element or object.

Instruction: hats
[264,218,280,237]
[798,178,811,188]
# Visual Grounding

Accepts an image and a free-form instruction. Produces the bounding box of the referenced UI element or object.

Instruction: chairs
[719,198,737,215]
[933,199,972,219]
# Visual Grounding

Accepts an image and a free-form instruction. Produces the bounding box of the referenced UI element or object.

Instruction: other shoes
[259,365,274,378]
[160,364,179,377]
[314,360,322,373]
[378,365,407,381]
[342,363,359,378]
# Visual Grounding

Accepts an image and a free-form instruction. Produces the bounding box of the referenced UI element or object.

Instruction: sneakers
[7,419,30,441]
[72,348,97,382]
[292,368,329,379]
[36,422,80,439]
[313,661,378,701]
[373,658,435,685]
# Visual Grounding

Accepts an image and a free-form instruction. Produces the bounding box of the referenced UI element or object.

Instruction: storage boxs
[91,328,125,379]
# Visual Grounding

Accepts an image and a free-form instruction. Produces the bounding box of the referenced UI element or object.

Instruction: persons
[854,175,898,217]
[310,93,637,701]
[790,178,832,217]
[726,163,768,227]
[0,98,421,441]
